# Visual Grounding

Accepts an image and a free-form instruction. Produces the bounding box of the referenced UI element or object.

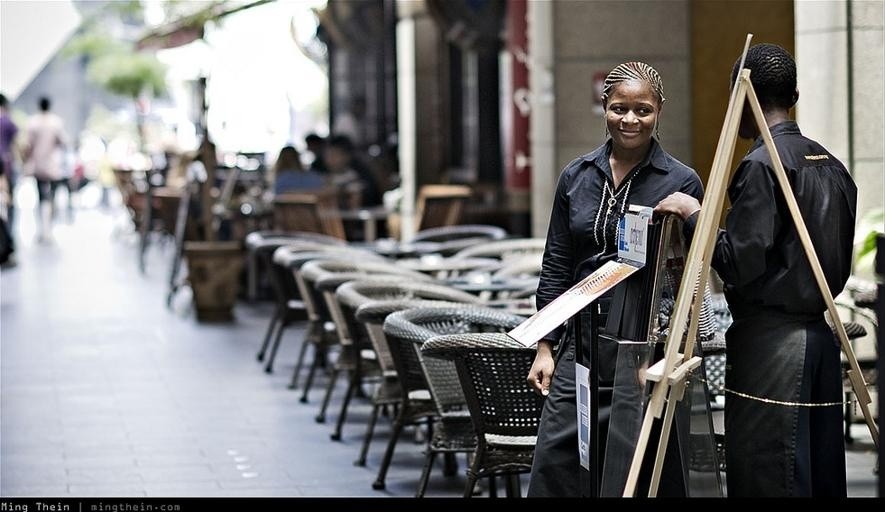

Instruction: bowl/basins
[604,168,643,208]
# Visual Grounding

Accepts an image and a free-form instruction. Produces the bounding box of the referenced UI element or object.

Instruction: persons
[523,59,708,499]
[261,91,386,243]
[17,95,67,249]
[652,37,859,500]
[0,93,19,224]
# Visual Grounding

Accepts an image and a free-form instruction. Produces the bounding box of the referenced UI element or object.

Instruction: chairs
[116,152,273,314]
[243,149,866,498]
